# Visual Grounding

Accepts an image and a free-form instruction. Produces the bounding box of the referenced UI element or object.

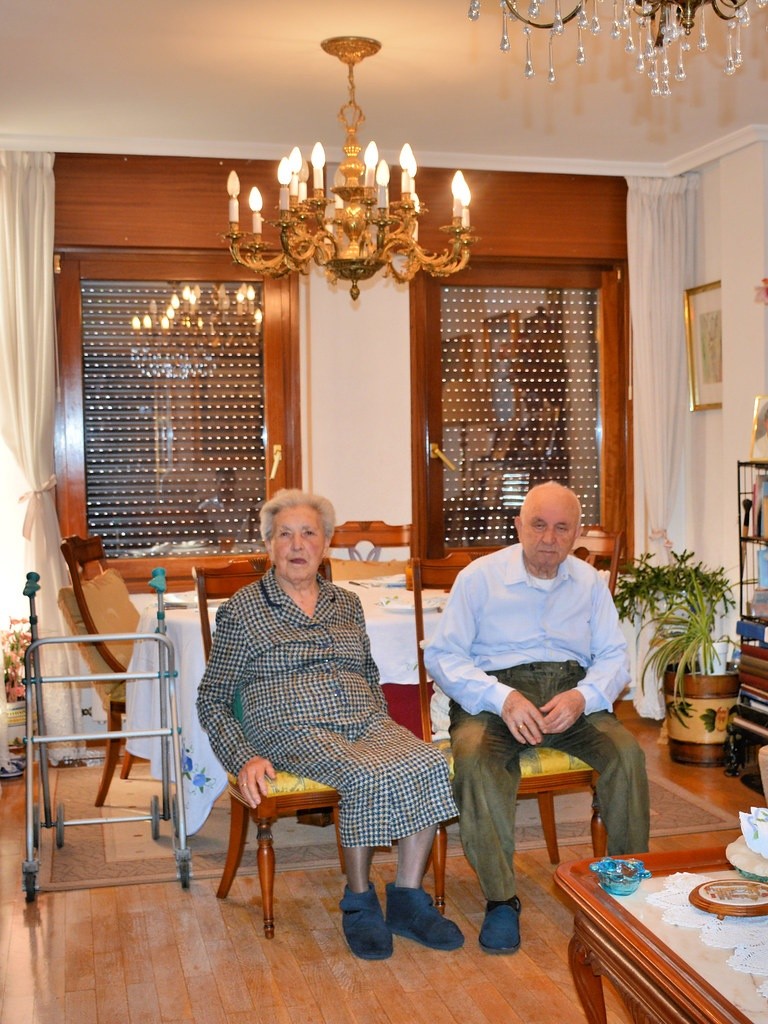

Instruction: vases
[0,700,32,779]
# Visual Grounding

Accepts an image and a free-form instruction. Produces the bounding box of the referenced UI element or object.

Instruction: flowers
[0,614,45,705]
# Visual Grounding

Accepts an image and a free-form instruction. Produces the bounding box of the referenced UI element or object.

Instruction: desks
[555,843,768,1024]
[104,581,451,832]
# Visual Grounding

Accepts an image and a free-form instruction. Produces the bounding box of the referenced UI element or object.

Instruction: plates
[162,589,199,604]
[372,573,445,612]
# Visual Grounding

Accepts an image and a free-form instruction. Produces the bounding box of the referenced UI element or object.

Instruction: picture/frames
[683,279,722,412]
[482,310,524,427]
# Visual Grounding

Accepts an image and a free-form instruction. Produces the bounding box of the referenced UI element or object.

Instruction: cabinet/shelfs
[722,459,768,783]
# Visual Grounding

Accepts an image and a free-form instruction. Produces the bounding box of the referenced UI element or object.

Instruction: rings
[518,724,524,730]
[241,783,247,787]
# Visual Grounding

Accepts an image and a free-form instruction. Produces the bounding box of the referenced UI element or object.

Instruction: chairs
[189,557,362,939]
[407,546,604,914]
[328,520,411,578]
[59,531,171,810]
[573,522,629,601]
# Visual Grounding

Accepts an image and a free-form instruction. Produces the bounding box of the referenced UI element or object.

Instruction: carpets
[24,761,739,890]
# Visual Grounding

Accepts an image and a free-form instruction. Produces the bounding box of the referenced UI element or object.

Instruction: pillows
[57,566,140,705]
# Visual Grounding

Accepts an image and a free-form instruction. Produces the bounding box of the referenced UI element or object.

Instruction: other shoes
[339,880,393,959]
[478,896,522,953]
[385,882,464,951]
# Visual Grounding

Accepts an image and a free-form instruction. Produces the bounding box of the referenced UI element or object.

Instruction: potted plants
[611,550,743,769]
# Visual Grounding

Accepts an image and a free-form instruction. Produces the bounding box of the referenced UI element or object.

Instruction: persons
[197,492,466,959]
[425,483,649,956]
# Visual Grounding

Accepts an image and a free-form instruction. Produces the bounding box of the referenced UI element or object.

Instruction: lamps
[217,32,485,308]
[441,381,496,503]
[126,276,266,375]
